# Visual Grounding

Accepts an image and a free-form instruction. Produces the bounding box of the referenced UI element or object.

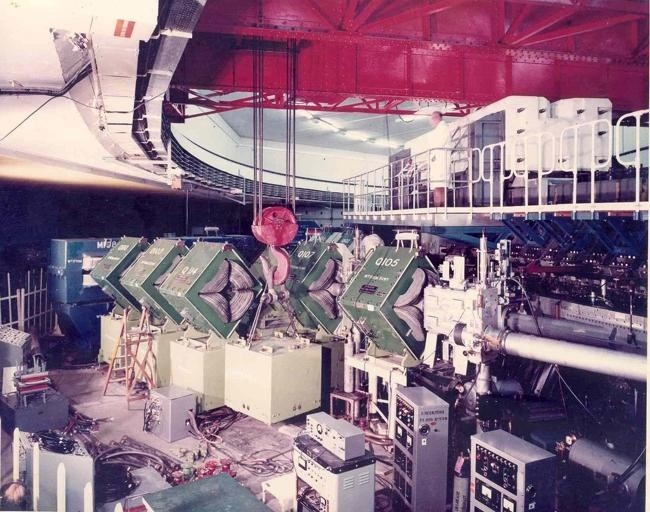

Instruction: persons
[429,111,453,208]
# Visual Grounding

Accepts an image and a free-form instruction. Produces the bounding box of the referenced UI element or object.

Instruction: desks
[328,390,370,431]
